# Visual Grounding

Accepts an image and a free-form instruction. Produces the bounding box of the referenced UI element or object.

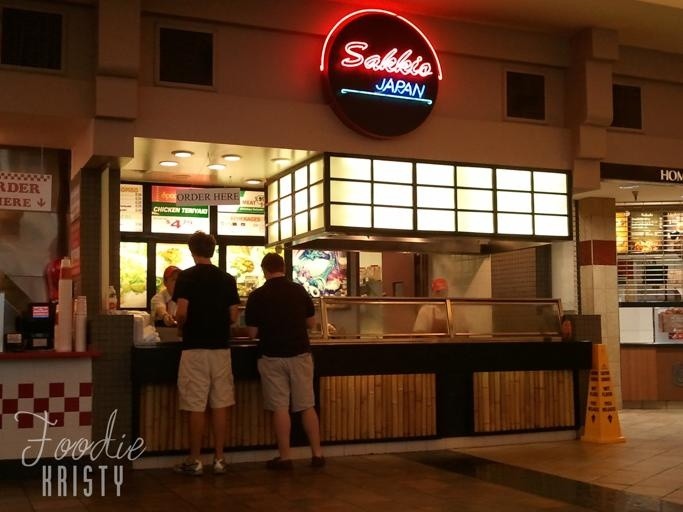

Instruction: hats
[432,278,448,291]
[164,266,182,279]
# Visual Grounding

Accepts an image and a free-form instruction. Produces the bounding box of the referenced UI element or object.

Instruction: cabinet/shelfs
[306,296,564,346]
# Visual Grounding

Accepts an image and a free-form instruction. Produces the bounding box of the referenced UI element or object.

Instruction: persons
[413,278,472,339]
[244,251,326,470]
[171,232,240,477]
[151,265,182,328]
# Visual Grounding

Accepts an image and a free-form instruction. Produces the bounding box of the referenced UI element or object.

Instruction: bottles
[109,285,117,313]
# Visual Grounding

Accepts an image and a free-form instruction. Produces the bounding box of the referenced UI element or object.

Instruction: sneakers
[268,457,293,466]
[312,456,327,467]
[173,457,227,476]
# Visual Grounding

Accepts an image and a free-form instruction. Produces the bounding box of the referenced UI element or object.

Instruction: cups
[53,259,88,352]
